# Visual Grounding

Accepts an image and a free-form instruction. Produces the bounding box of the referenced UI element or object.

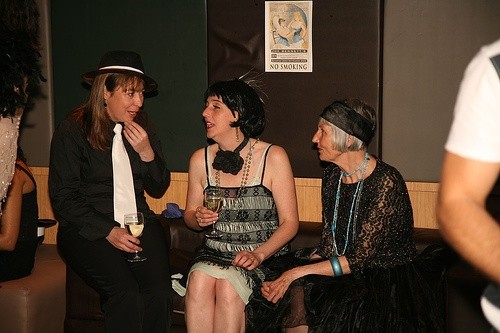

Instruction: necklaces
[212,137,260,225]
[211,134,250,177]
[331,150,370,257]
[342,152,369,177]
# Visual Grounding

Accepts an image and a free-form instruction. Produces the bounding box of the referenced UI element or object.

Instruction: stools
[0,243,64,333]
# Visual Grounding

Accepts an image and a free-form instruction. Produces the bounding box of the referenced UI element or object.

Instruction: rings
[200,219,202,223]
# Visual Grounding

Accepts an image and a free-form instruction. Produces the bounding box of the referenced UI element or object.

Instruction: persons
[243,96,447,333]
[183,65,300,333]
[48,51,175,333]
[0,22,47,221]
[0,144,38,282]
[435,40,500,333]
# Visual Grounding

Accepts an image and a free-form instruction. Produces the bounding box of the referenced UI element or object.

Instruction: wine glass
[124,212,145,262]
[204,189,224,238]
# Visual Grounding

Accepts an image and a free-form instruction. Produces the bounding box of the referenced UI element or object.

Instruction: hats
[81,50,158,97]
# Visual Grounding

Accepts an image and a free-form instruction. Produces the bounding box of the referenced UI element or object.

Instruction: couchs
[64,213,442,333]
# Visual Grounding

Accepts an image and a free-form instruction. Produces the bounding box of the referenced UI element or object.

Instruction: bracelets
[328,256,343,277]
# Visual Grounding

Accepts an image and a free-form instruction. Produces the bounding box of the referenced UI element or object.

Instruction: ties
[111,124,137,227]
